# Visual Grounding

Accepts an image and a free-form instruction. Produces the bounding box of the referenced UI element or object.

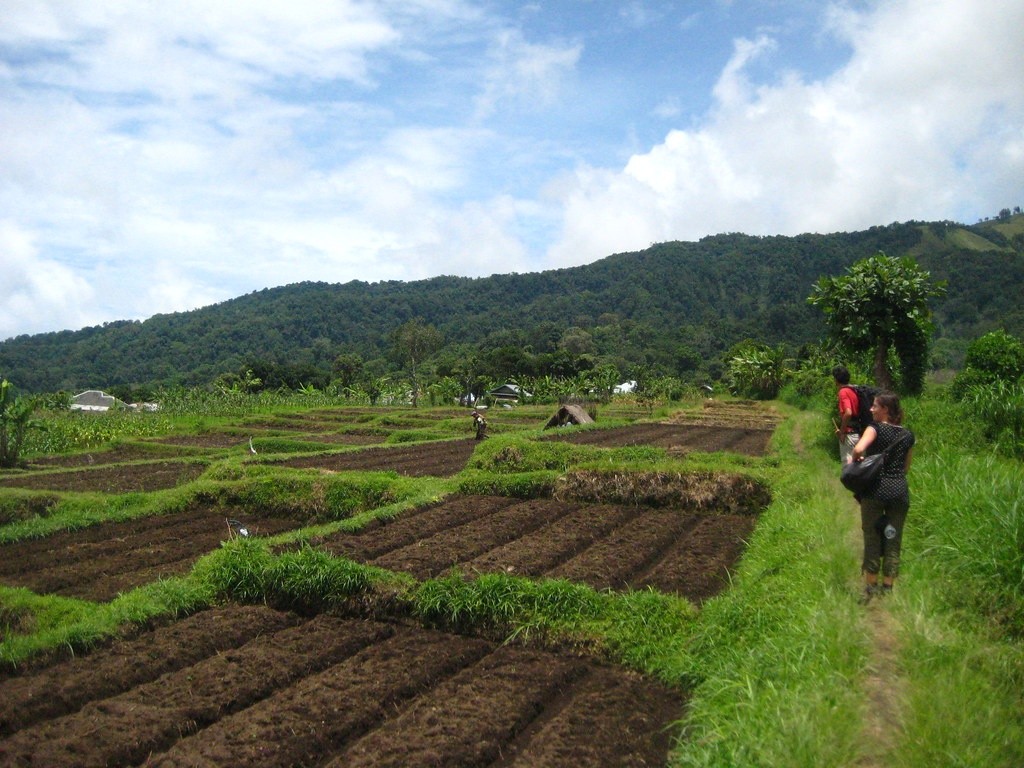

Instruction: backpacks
[839,384,877,437]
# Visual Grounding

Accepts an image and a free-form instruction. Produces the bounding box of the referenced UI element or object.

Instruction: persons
[832,366,877,466]
[471,411,488,441]
[840,390,915,606]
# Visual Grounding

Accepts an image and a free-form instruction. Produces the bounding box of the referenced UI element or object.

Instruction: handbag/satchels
[840,430,912,496]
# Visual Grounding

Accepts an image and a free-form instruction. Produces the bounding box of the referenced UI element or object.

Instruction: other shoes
[856,583,894,607]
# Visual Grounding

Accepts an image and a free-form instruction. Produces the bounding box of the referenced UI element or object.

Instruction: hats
[470,411,477,416]
